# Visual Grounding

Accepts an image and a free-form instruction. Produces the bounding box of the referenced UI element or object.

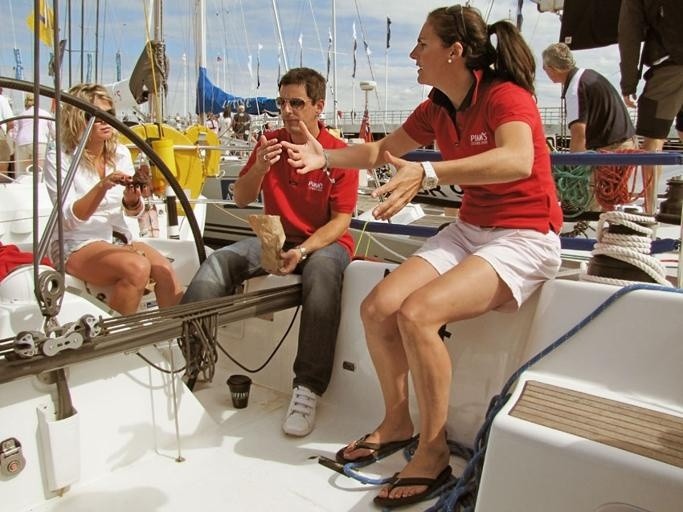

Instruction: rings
[263,155,268,161]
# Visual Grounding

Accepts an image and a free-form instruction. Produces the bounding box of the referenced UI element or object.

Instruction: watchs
[295,245,308,263]
[421,161,439,191]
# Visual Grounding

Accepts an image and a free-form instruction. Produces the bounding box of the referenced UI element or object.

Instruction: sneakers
[282,385,321,437]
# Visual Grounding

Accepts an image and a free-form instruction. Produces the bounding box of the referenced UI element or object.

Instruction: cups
[227,374,252,408]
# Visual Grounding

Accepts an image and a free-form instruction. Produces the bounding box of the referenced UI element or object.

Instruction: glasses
[86,109,115,123]
[275,97,311,111]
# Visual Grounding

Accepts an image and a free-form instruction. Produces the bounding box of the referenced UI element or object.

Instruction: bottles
[137,193,159,238]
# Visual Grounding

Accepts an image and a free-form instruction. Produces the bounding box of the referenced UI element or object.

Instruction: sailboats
[0,0,683,282]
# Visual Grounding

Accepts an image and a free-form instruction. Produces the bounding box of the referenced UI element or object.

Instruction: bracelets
[321,150,328,170]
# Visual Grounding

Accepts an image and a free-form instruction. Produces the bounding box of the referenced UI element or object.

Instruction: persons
[173,67,360,438]
[44,83,184,320]
[616,0,683,217]
[540,42,639,212]
[279,5,564,506]
[174,105,274,142]
[0,86,15,184]
[12,93,56,179]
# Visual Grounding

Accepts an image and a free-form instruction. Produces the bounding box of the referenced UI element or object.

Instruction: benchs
[471,278,682,511]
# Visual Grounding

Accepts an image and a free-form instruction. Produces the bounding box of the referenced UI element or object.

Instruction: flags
[27,0,54,48]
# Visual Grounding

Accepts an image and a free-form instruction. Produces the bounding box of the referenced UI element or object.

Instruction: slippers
[337,433,414,464]
[374,465,452,507]
